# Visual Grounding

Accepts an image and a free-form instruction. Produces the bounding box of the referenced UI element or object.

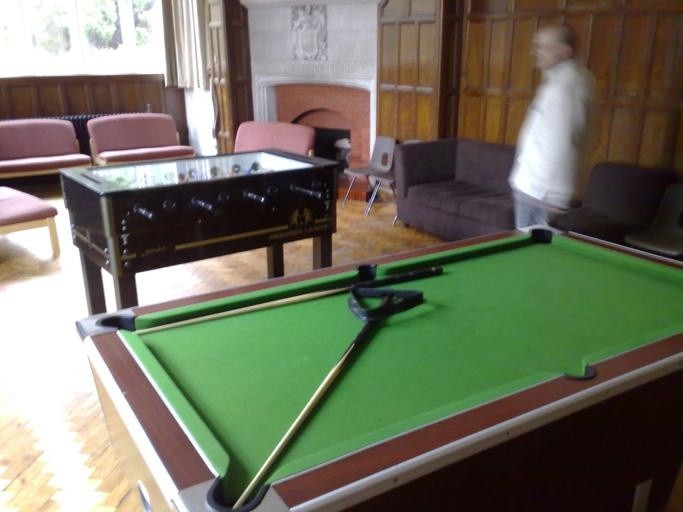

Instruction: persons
[508,23,595,226]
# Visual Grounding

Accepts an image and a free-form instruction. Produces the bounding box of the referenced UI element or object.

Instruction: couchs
[391,133,582,243]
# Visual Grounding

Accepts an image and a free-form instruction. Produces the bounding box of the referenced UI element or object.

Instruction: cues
[232,295,393,509]
[134,266,442,335]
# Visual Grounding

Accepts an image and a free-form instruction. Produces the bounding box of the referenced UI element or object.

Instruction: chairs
[365,139,423,225]
[556,163,683,260]
[233,120,314,157]
[86,113,195,169]
[340,135,394,211]
[0,182,60,261]
[0,120,91,191]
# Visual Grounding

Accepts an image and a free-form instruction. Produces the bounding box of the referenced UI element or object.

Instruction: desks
[74,220,680,512]
